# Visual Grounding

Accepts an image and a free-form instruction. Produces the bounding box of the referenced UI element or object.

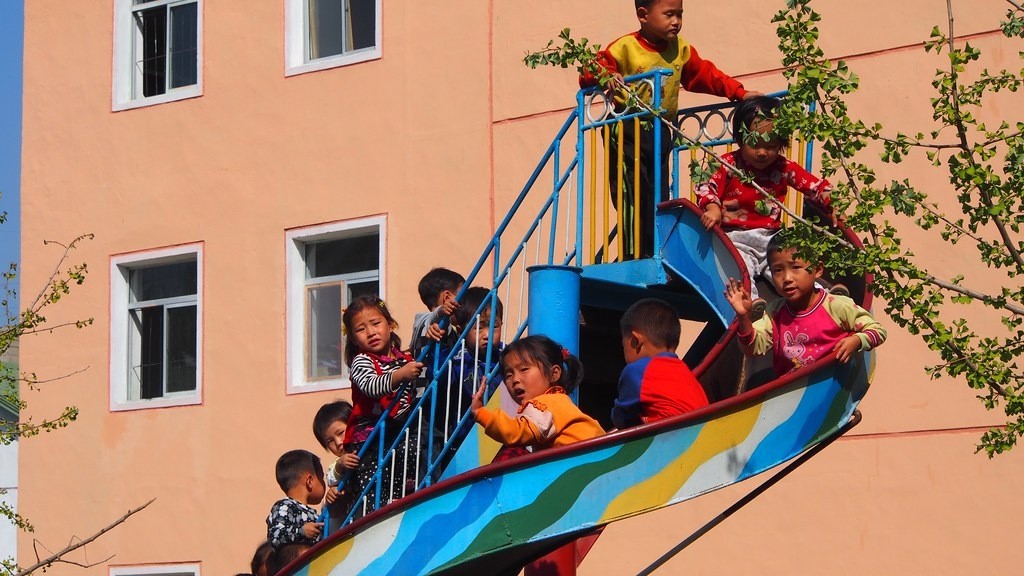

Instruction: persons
[611,298,710,429]
[579,0,766,258]
[724,229,888,378]
[694,95,850,322]
[238,266,510,576]
[473,334,606,463]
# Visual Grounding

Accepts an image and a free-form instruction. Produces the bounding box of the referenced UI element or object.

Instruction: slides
[276,189,872,576]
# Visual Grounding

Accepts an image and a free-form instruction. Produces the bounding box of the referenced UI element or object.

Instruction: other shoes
[751,297,768,322]
[828,284,851,297]
